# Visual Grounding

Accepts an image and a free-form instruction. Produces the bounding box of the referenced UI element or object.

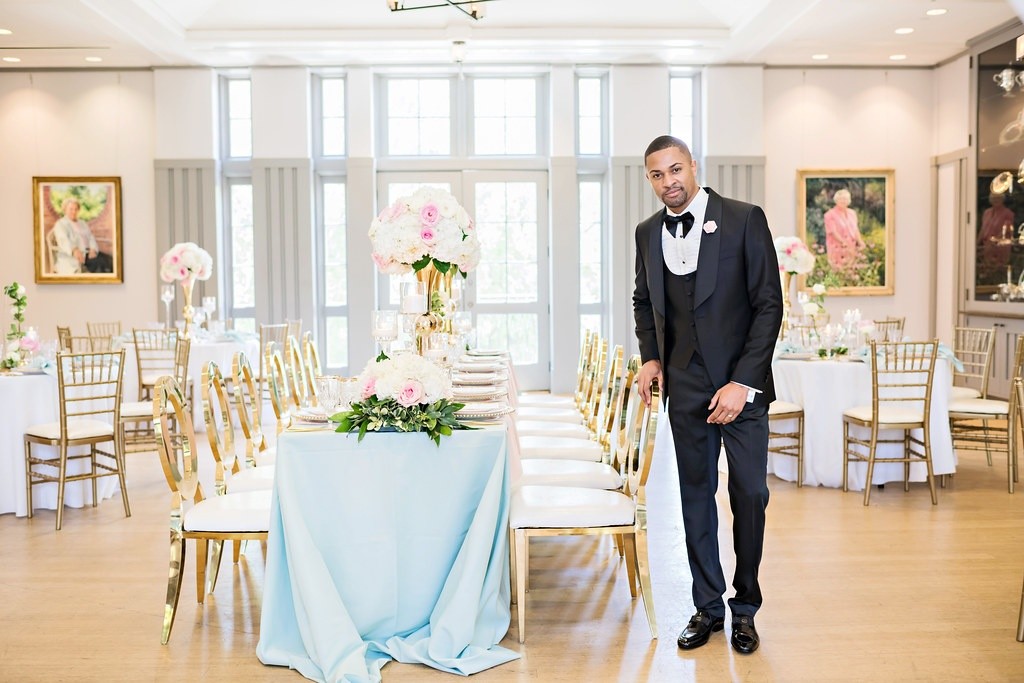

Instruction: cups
[149,322,224,341]
[0,328,57,366]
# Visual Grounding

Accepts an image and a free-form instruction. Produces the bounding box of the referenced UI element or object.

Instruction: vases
[179,279,195,340]
[415,260,458,356]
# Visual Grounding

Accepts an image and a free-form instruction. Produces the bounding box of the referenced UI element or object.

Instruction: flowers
[773,236,816,275]
[1,281,39,367]
[159,241,214,282]
[327,351,466,448]
[367,185,482,276]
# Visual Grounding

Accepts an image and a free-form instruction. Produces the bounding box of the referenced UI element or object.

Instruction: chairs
[21,328,356,640]
[767,316,1024,506]
[511,329,660,643]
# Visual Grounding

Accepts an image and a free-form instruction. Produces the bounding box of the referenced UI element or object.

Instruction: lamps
[449,40,468,81]
[387,0,499,20]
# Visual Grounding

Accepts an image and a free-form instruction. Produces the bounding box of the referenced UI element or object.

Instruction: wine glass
[161,284,174,333]
[399,282,427,354]
[372,310,398,359]
[193,307,205,327]
[776,310,876,349]
[202,297,216,322]
[798,291,810,317]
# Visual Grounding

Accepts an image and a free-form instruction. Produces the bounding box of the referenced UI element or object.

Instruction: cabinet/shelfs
[959,17,1024,402]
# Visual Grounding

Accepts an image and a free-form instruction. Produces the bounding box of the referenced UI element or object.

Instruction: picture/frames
[32,175,125,285]
[794,166,896,298]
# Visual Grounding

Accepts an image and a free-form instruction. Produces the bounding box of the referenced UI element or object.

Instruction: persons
[53,198,99,274]
[632,135,783,654]
[823,189,866,268]
[978,190,1014,265]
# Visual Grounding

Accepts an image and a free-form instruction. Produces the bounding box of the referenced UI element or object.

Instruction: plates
[291,406,328,422]
[449,348,515,419]
[778,353,812,360]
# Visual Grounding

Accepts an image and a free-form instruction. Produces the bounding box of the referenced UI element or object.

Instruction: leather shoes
[731,615,759,654]
[677,610,724,649]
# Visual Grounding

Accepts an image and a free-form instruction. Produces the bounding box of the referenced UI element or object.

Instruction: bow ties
[663,211,695,238]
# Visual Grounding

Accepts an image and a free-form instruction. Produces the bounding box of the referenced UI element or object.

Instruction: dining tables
[257,353,522,683]
[111,331,262,430]
[718,341,952,491]
[0,350,128,517]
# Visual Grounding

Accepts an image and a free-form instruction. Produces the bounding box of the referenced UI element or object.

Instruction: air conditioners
[777,272,792,341]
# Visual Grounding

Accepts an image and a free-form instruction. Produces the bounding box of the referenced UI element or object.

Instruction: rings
[728,414,733,417]
